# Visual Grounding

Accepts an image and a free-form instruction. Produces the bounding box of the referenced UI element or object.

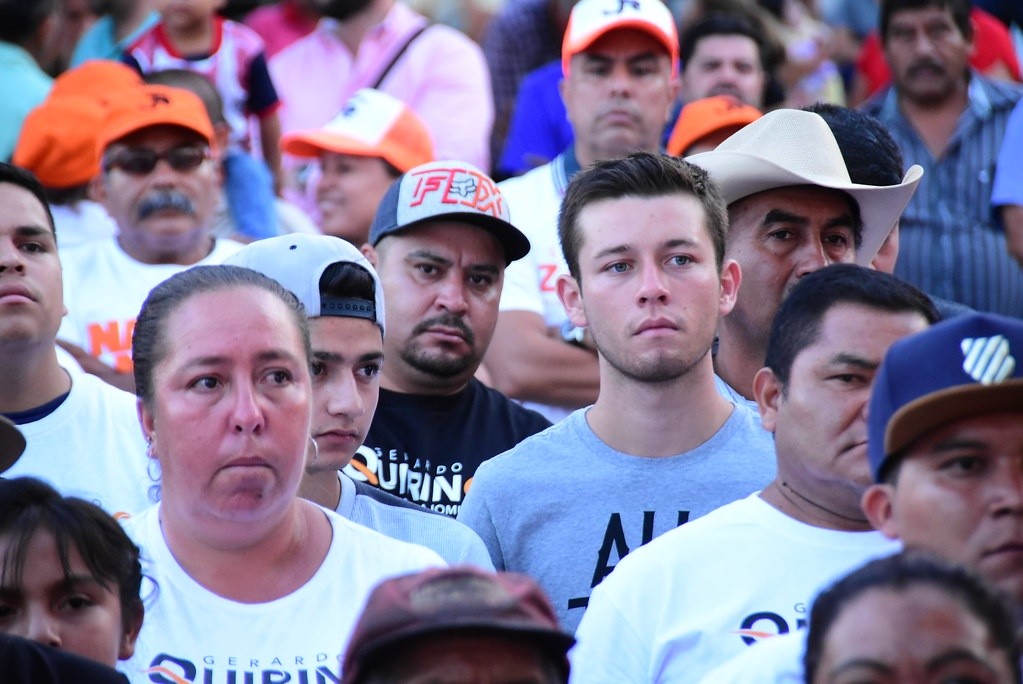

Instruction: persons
[0,0,1023,399]
[459,150,778,634]
[348,162,558,518]
[0,162,162,522]
[803,552,1023,684]
[707,311,1023,684]
[341,567,576,684]
[687,111,925,415]
[112,265,446,683]
[224,231,497,572]
[568,266,941,684]
[0,415,145,684]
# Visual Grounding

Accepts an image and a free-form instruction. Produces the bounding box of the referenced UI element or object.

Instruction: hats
[219,232,386,342]
[666,94,763,158]
[340,565,578,684]
[560,0,679,78]
[866,310,1023,487]
[681,108,924,267]
[367,159,530,270]
[278,87,435,173]
[94,85,217,172]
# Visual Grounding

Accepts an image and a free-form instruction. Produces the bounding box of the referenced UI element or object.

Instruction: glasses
[101,142,214,175]
